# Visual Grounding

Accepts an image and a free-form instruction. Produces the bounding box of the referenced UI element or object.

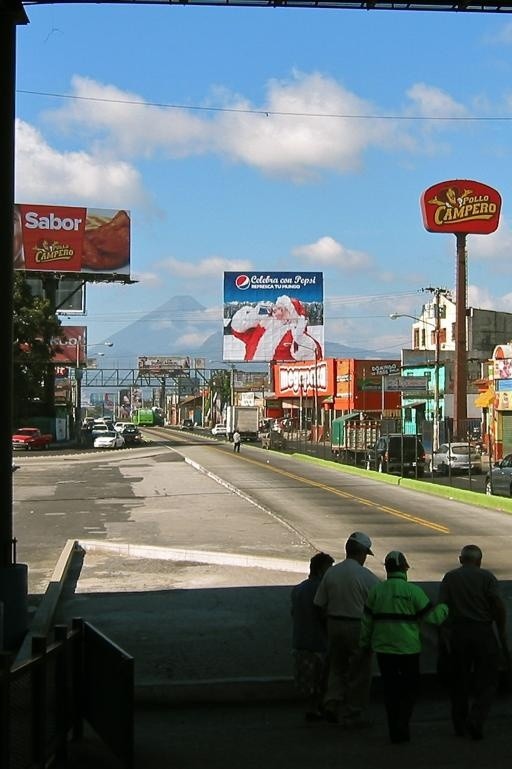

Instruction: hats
[349,531,374,557]
[384,550,411,569]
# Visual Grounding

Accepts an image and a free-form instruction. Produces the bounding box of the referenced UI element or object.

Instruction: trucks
[328,412,397,469]
[225,405,260,442]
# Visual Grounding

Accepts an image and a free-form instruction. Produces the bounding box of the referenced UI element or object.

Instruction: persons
[231,294,322,360]
[232,429,241,453]
[313,531,382,729]
[14,205,25,269]
[291,552,336,722]
[438,545,509,742]
[358,550,449,745]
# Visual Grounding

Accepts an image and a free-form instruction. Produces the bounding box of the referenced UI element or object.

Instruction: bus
[130,407,155,427]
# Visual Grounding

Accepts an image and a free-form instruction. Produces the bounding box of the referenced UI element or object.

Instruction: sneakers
[234,451,241,453]
[305,705,484,744]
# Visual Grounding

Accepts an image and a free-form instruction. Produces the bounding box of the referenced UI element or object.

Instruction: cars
[483,454,512,499]
[212,423,227,437]
[258,414,313,436]
[424,441,482,477]
[80,415,144,449]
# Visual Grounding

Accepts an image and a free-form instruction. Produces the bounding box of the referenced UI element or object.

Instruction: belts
[328,615,361,622]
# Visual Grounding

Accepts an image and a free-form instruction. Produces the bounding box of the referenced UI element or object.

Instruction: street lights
[386,311,443,476]
[75,341,119,415]
[209,359,239,412]
[283,340,321,445]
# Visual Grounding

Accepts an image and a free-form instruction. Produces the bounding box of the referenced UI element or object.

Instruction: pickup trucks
[11,426,53,451]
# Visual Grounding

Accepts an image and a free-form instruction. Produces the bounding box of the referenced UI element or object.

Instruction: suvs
[363,430,427,476]
[180,419,194,430]
